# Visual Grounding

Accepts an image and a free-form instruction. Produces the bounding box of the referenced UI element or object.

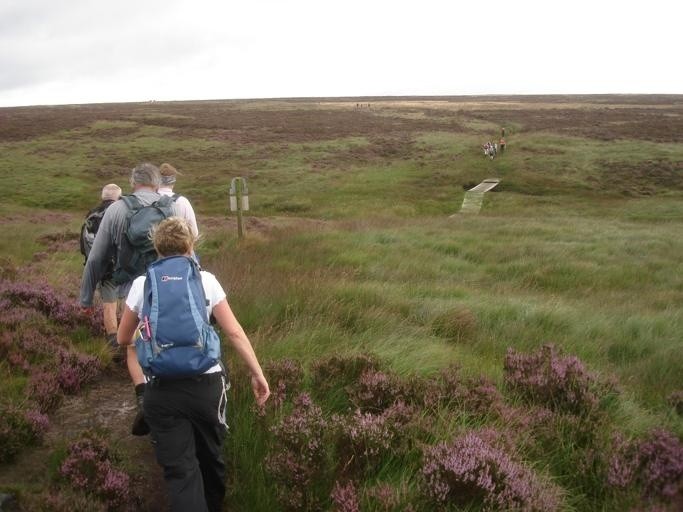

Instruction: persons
[480,125,510,162]
[79,180,126,353]
[115,213,270,512]
[77,162,201,437]
[156,162,201,267]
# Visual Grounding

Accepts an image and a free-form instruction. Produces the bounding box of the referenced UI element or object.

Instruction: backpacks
[121,195,174,275]
[83,209,106,258]
[135,256,220,376]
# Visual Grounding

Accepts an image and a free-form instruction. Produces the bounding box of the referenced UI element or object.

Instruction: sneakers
[131,401,146,436]
